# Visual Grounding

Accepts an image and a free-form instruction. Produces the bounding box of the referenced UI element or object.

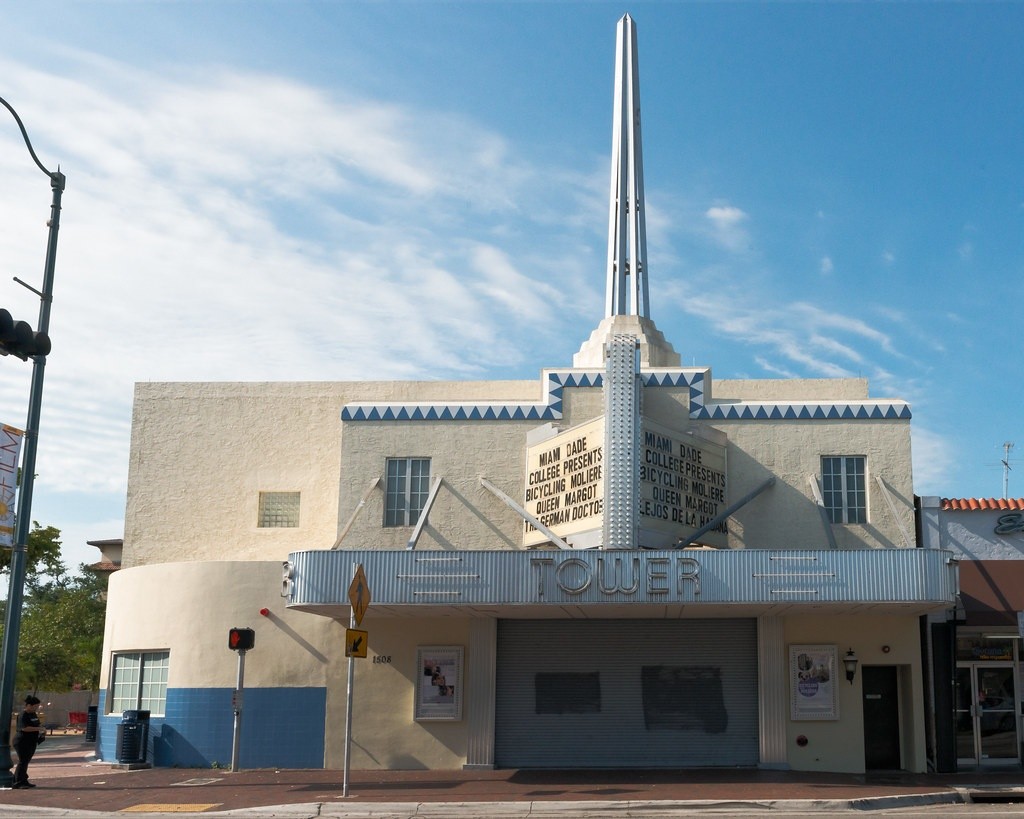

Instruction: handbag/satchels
[38,732,45,745]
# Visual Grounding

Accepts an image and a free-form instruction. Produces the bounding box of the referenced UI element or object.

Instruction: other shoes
[12,780,36,789]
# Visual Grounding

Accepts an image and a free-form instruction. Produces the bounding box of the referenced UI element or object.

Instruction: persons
[11,694,47,790]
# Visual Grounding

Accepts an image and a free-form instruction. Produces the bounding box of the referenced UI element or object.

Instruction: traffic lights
[229,628,254,650]
[0,308,52,361]
